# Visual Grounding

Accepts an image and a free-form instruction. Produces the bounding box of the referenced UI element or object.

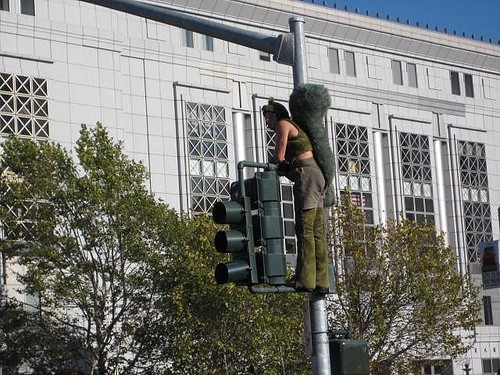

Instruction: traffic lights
[212,196,260,285]
[230,171,288,287]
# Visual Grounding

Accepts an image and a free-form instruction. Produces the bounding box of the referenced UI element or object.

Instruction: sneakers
[314,286,329,294]
[286,278,314,293]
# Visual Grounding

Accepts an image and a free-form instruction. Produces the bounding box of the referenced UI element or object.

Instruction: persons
[262,102,329,295]
